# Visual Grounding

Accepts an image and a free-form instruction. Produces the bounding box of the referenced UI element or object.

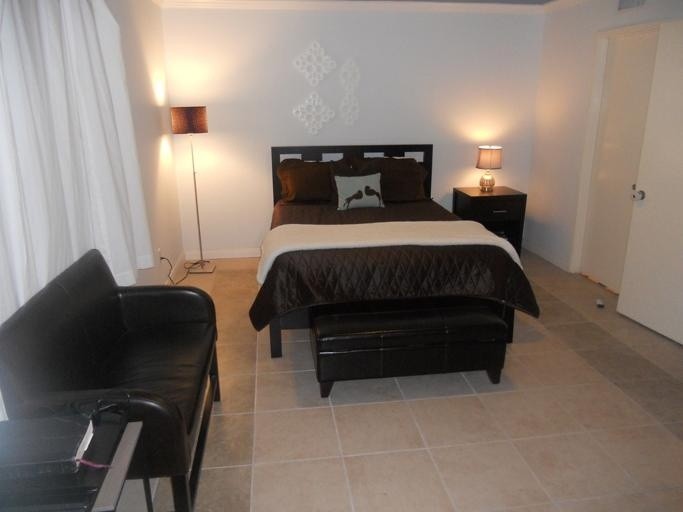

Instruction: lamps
[170,106,216,273]
[474,145,503,193]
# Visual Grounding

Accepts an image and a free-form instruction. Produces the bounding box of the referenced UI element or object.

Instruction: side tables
[0,420,155,512]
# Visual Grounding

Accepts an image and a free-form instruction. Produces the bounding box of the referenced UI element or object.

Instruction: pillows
[353,158,418,203]
[330,165,387,211]
[414,163,433,201]
[277,155,357,206]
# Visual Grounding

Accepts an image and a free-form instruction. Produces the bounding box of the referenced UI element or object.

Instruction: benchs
[307,295,508,398]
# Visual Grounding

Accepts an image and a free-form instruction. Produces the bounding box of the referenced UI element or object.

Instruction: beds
[250,145,539,359]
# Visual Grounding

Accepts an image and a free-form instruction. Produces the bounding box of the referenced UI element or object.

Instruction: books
[0,415,94,483]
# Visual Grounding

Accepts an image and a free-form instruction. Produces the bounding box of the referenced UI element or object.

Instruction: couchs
[0,248,220,512]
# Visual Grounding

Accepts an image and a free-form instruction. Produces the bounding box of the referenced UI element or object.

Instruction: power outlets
[157,253,170,266]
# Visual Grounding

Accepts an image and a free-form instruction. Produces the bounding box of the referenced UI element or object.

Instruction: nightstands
[451,186,527,258]
